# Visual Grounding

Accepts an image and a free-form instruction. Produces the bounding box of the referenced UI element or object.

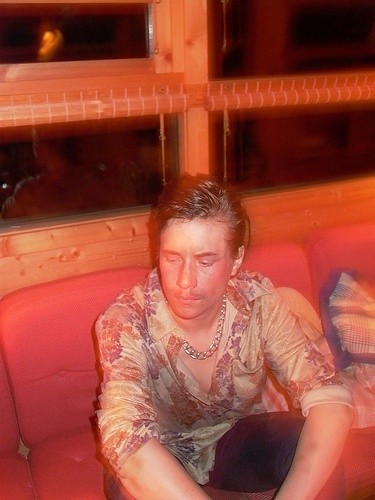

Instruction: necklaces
[164,294,228,361]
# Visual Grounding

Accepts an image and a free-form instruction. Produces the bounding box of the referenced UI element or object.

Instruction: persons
[93,174,354,500]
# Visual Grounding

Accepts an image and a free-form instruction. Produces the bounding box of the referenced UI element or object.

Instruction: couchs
[1,220,374,500]
